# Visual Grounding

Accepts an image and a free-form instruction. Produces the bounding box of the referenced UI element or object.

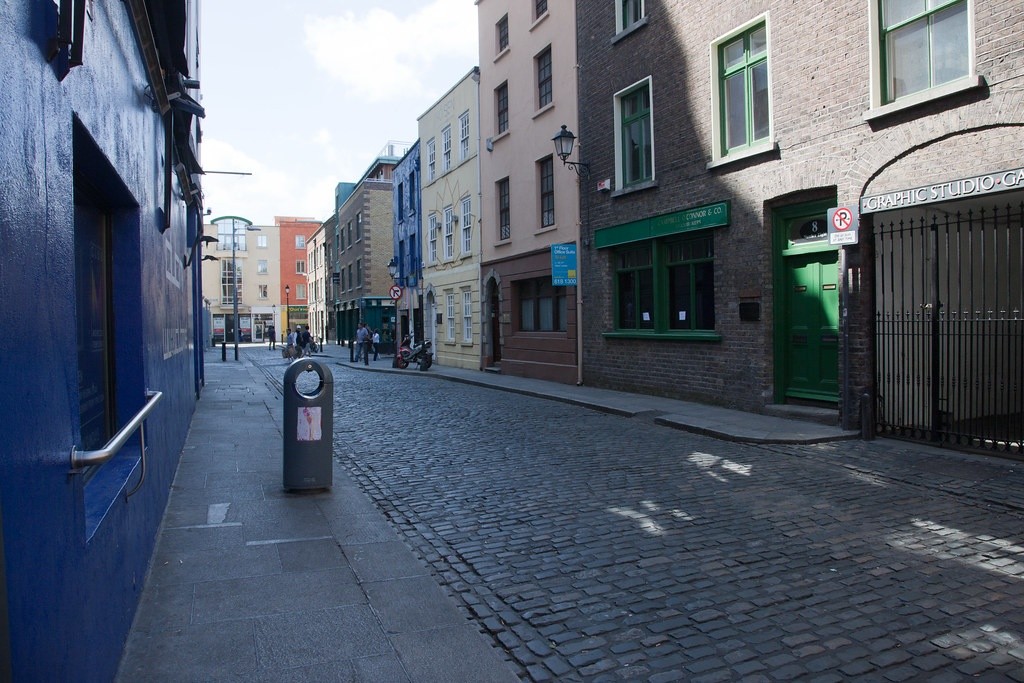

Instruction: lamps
[183,236,219,268]
[551,124,591,179]
[202,254,218,262]
[387,260,409,286]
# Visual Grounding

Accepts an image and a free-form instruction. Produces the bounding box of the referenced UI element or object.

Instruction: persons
[293,325,303,359]
[302,326,312,357]
[353,323,368,362]
[364,323,371,344]
[285,328,294,364]
[372,328,380,361]
[268,326,276,350]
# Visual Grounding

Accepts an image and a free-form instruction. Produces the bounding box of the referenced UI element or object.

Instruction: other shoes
[374,359,376,361]
[353,360,358,362]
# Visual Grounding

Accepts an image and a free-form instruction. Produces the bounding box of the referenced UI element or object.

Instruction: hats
[297,324,302,329]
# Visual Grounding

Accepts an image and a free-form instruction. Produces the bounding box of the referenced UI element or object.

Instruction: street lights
[284,285,293,351]
[234,228,262,361]
[272,304,276,326]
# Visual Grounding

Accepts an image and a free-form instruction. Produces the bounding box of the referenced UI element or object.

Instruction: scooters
[396,330,434,371]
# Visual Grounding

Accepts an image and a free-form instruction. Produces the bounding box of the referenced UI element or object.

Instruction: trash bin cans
[284,356,334,493]
